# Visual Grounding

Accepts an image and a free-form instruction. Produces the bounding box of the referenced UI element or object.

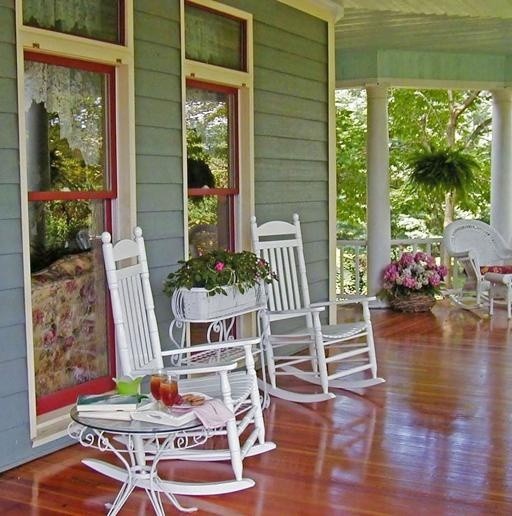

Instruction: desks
[67,388,222,516]
[484,272,512,318]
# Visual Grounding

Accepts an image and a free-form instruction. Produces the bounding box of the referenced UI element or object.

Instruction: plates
[174,391,214,409]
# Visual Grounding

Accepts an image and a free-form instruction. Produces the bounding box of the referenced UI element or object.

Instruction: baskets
[391,295,436,313]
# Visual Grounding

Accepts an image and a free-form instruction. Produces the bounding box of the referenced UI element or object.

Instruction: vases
[388,291,437,313]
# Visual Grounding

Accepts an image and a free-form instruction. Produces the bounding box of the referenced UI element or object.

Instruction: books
[76,392,213,427]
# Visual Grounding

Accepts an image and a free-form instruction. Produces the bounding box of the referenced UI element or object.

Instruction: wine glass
[160,373,178,415]
[151,369,168,411]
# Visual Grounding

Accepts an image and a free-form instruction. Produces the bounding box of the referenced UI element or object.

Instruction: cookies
[181,394,204,407]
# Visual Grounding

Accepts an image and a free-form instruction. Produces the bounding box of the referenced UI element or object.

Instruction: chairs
[441,219,512,320]
[81,227,277,495]
[249,213,385,402]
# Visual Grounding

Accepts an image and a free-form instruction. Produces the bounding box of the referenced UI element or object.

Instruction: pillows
[462,266,511,275]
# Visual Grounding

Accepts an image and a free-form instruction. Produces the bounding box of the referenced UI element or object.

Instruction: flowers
[375,251,448,295]
[162,249,280,298]
